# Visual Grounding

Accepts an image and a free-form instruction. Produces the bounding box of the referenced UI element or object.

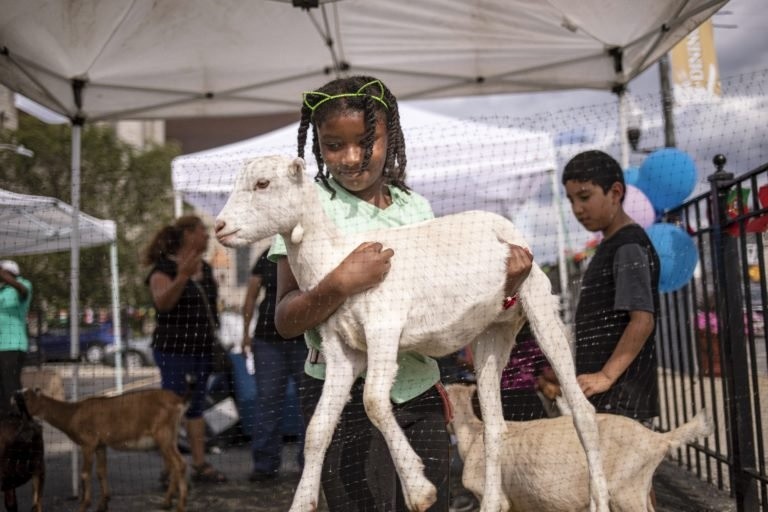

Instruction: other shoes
[252,469,282,486]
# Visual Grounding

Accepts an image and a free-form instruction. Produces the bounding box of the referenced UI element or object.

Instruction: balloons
[620,165,641,184]
[642,147,698,213]
[643,221,699,291]
[618,183,655,228]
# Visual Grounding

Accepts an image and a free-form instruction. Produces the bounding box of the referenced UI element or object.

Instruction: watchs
[503,293,519,310]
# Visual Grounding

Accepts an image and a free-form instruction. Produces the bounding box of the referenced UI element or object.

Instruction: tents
[1,190,126,497]
[169,86,645,351]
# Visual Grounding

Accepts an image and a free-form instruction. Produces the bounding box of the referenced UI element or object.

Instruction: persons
[242,245,313,481]
[146,214,236,489]
[277,74,537,512]
[0,260,34,396]
[556,151,660,434]
[489,314,561,421]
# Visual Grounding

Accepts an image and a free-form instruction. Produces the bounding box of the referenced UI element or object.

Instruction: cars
[104,334,157,370]
[27,318,113,365]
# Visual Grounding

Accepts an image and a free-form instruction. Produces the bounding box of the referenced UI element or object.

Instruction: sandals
[160,471,171,489]
[191,462,228,483]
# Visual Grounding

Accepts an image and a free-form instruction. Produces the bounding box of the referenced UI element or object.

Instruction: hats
[0,259,20,275]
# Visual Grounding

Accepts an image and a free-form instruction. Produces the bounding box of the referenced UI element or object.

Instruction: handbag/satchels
[210,336,234,373]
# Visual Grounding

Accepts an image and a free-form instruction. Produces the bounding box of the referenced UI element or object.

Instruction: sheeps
[439,384,718,512]
[213,155,610,512]
[11,386,192,512]
